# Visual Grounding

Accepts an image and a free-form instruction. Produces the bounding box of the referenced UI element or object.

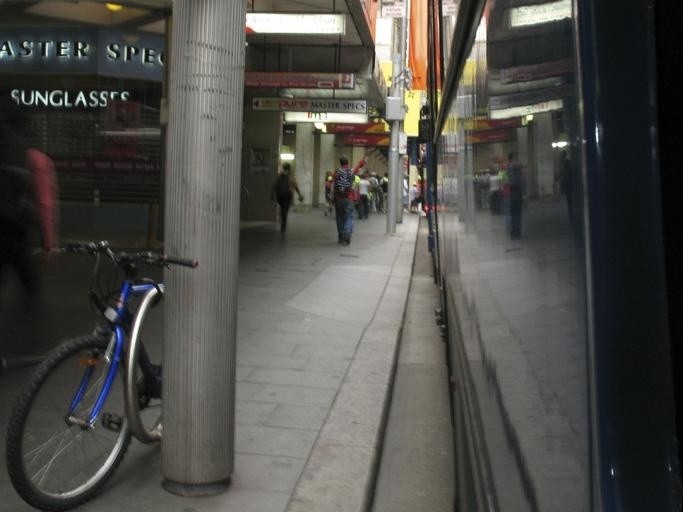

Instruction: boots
[338,232,351,243]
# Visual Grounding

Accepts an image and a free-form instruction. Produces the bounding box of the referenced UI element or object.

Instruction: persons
[270,161,304,235]
[2,105,67,358]
[330,153,371,245]
[322,166,423,220]
[434,162,514,217]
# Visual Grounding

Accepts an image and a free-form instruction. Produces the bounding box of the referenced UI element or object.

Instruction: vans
[67,123,162,168]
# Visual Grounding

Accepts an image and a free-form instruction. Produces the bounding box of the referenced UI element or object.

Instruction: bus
[415,0,683,512]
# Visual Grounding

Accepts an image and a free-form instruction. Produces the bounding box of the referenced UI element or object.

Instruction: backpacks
[333,168,353,194]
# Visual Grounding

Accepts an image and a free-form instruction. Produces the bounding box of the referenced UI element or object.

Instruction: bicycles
[2,236,198,512]
